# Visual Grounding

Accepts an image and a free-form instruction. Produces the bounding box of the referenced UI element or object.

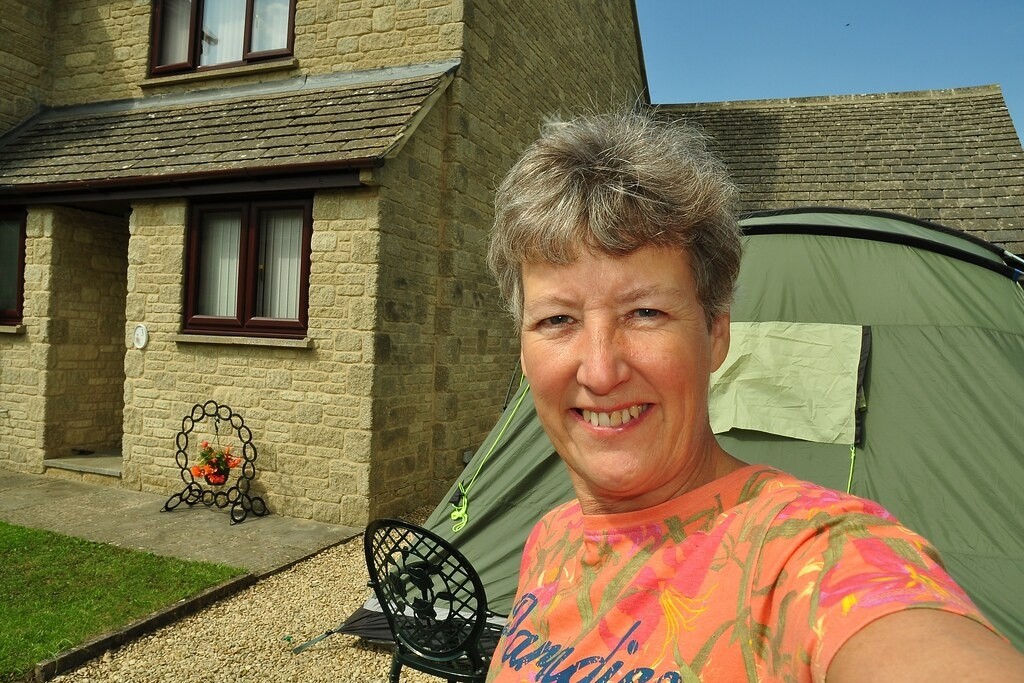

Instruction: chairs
[365,516,505,683]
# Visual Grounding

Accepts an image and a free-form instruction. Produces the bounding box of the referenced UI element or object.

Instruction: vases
[202,468,231,485]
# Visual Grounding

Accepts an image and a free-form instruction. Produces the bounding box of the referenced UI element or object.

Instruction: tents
[294,205,1022,656]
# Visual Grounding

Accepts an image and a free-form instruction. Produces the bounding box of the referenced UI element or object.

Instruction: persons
[486,87,1024,683]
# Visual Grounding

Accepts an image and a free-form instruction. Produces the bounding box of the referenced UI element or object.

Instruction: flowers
[191,441,242,479]
